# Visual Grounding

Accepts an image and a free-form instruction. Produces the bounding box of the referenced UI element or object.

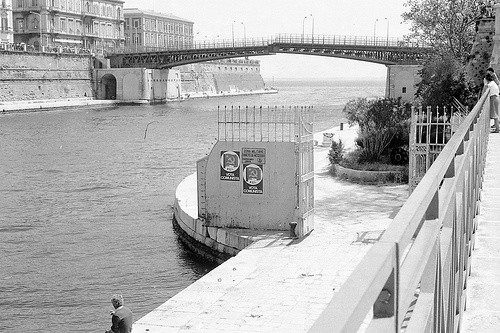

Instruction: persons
[484,74,500,133]
[104,293,133,333]
[486,67,500,128]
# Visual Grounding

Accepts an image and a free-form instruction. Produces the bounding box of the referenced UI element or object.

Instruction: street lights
[232,21,236,50]
[128,23,143,53]
[310,14,314,46]
[205,36,207,51]
[374,18,378,47]
[303,17,307,45]
[241,22,246,49]
[197,32,199,51]
[385,18,389,51]
